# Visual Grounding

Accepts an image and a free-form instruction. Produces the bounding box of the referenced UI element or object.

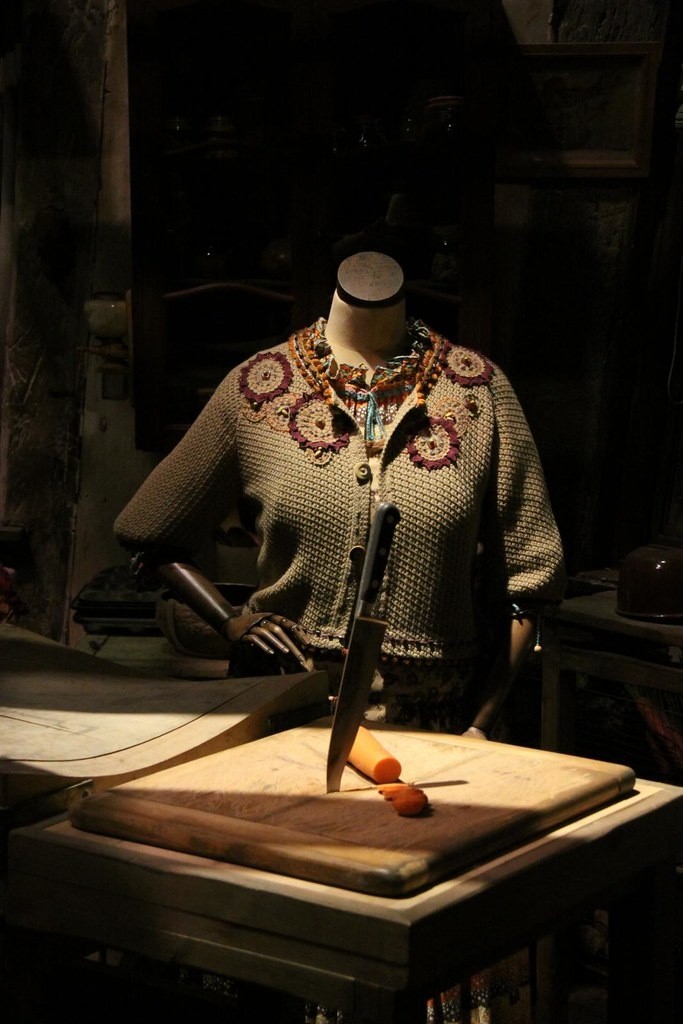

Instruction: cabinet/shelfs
[129,1,502,450]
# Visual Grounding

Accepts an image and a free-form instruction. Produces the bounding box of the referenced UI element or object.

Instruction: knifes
[325,499,401,793]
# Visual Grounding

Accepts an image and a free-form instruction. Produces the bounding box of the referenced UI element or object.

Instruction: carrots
[346,724,430,817]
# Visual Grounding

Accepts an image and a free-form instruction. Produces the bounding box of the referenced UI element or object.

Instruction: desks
[7,779,683,1024]
[531,587,683,1024]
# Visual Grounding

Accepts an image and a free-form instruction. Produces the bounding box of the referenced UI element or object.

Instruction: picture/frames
[482,41,658,179]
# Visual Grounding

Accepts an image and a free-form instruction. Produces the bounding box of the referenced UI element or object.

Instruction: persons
[112,253,566,749]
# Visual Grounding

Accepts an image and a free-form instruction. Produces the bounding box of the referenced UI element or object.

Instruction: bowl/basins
[613,542,683,625]
